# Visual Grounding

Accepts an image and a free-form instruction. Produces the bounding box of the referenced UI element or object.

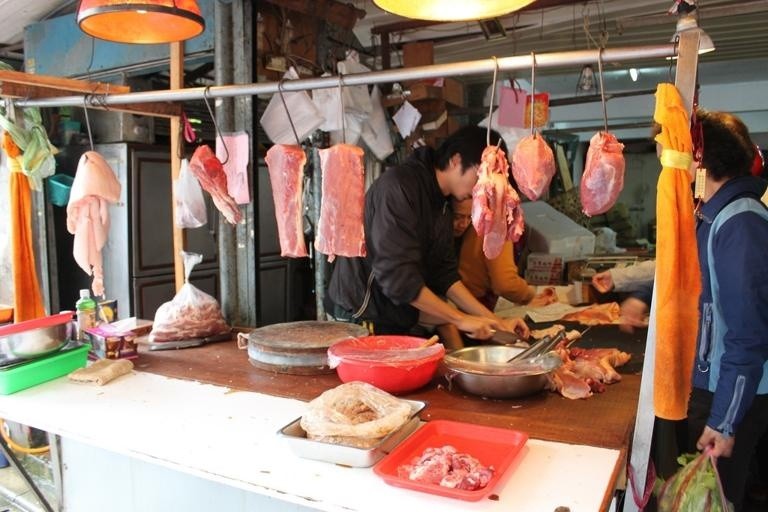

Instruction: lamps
[371,0,536,22]
[74,0,206,45]
[665,0,717,60]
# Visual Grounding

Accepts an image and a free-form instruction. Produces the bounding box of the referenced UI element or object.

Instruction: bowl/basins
[326,335,562,398]
[0,319,76,365]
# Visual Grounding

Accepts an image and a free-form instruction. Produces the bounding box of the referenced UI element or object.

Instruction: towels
[2,131,48,324]
[651,81,702,424]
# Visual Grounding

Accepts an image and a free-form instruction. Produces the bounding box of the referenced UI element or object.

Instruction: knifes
[149,333,233,351]
[485,329,519,345]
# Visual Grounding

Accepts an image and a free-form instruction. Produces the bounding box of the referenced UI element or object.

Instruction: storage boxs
[520,200,597,262]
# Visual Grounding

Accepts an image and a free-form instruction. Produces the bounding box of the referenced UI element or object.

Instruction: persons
[590,107,768,512]
[405,194,477,351]
[324,127,532,349]
[459,219,558,310]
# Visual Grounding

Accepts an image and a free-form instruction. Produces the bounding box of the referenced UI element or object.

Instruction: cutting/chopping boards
[248,320,369,374]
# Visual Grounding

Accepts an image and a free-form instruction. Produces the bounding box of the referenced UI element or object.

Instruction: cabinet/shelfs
[89,139,318,325]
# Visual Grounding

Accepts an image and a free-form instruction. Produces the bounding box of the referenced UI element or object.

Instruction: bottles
[75,289,97,342]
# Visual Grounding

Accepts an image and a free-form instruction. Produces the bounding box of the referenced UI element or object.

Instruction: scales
[579,256,653,277]
